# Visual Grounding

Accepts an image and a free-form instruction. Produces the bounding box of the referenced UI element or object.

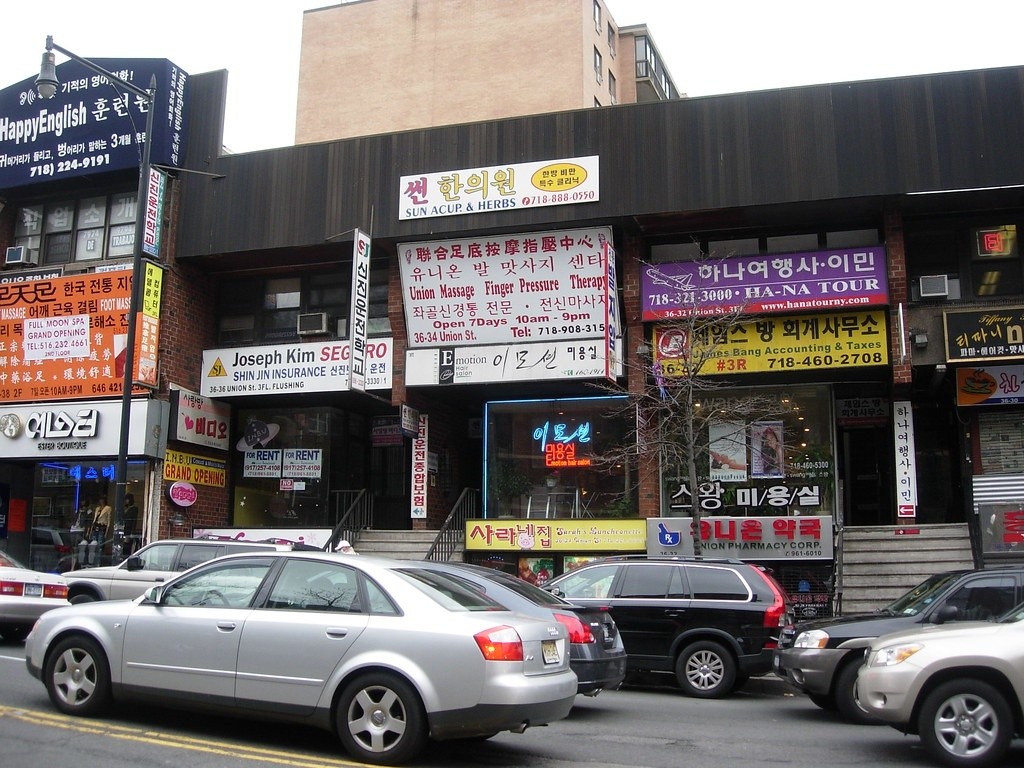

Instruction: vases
[547,478,557,487]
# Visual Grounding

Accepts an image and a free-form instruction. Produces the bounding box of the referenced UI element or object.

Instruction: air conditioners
[919,275,949,300]
[296,312,334,335]
[5,246,32,263]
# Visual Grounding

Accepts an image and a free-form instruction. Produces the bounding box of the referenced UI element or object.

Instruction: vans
[61,538,305,605]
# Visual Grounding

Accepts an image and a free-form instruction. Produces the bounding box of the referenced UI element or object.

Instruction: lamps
[935,365,947,373]
[170,514,185,526]
[636,345,649,359]
[914,334,928,348]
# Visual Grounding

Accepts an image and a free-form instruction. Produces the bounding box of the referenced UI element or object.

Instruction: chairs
[554,494,573,518]
[582,490,602,519]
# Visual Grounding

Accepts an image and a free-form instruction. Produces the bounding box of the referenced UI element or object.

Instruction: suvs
[536,557,795,698]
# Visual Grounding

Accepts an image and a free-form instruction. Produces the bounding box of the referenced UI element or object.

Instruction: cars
[24,552,579,768]
[30,527,84,573]
[772,566,1024,726]
[0,550,73,641]
[854,602,1024,768]
[308,560,628,698]
[55,535,143,575]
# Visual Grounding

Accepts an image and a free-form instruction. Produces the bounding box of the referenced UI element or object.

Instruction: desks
[548,493,576,519]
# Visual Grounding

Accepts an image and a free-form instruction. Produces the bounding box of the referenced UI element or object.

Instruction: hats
[335,540,350,550]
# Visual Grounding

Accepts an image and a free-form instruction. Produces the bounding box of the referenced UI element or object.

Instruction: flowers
[545,469,559,480]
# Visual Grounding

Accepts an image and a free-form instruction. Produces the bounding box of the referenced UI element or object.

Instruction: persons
[334,540,358,556]
[797,580,813,621]
[759,429,781,476]
[70,492,138,571]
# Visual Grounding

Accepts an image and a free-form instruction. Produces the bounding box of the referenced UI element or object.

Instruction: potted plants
[797,450,833,515]
[664,456,712,518]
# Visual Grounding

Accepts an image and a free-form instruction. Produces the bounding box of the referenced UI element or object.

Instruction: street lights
[35,36,157,568]
